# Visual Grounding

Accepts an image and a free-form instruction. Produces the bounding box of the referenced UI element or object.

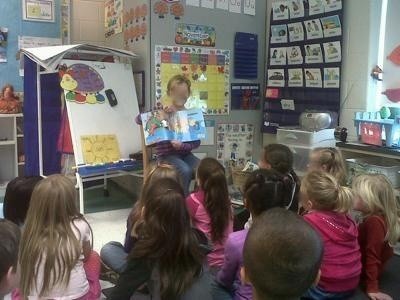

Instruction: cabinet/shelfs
[276,127,335,181]
[0,113,25,200]
[336,142,400,197]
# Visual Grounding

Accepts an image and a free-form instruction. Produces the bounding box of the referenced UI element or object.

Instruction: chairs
[140,124,157,184]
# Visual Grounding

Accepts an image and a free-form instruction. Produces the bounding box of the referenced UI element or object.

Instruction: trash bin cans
[231,161,260,193]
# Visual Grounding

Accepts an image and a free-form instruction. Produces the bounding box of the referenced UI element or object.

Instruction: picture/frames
[22,0,55,23]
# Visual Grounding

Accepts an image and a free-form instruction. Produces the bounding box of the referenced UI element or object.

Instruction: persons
[135,74,201,197]
[100,142,400,299]
[0,174,101,300]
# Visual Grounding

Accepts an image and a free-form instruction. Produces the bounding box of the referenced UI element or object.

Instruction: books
[140,108,208,146]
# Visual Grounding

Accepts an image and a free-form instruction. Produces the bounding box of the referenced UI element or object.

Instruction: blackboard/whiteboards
[59,58,142,167]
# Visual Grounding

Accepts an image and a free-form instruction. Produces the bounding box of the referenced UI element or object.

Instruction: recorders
[299,109,338,132]
